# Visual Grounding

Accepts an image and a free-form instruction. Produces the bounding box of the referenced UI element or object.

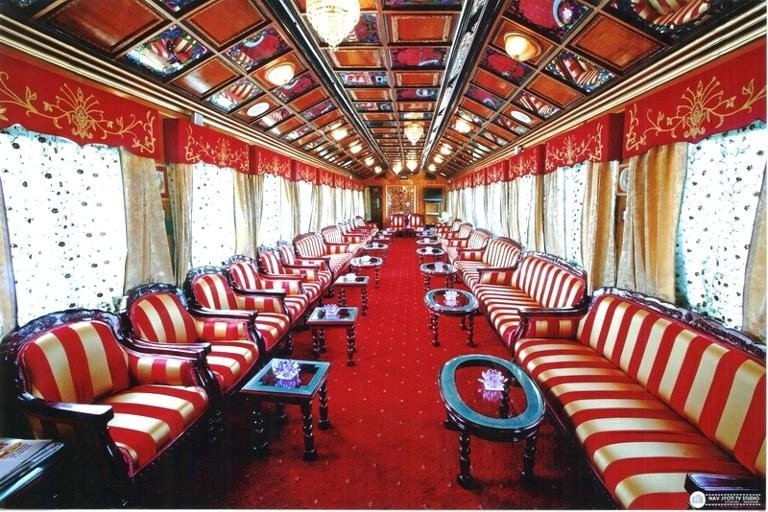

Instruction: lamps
[300,1,370,51]
[503,30,542,63]
[264,62,296,86]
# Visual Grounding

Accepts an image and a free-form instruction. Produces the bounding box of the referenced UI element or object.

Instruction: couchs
[1,215,391,510]
[418,207,765,509]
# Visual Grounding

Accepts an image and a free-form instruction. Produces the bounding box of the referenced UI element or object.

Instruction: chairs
[407,213,425,237]
[390,212,406,237]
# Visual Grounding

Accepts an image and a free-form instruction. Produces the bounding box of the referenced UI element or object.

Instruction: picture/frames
[385,185,416,219]
